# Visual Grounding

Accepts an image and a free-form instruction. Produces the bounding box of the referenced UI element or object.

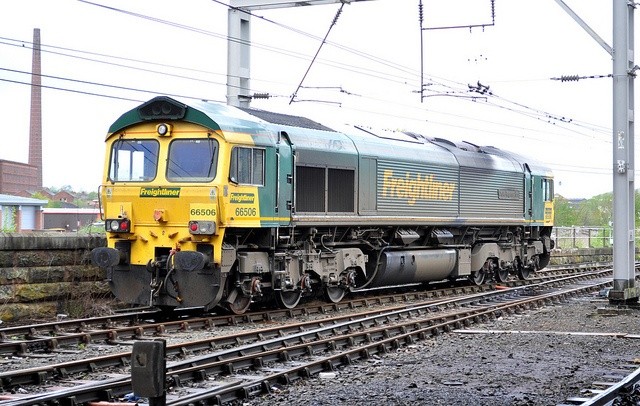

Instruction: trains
[92,95,555,315]
